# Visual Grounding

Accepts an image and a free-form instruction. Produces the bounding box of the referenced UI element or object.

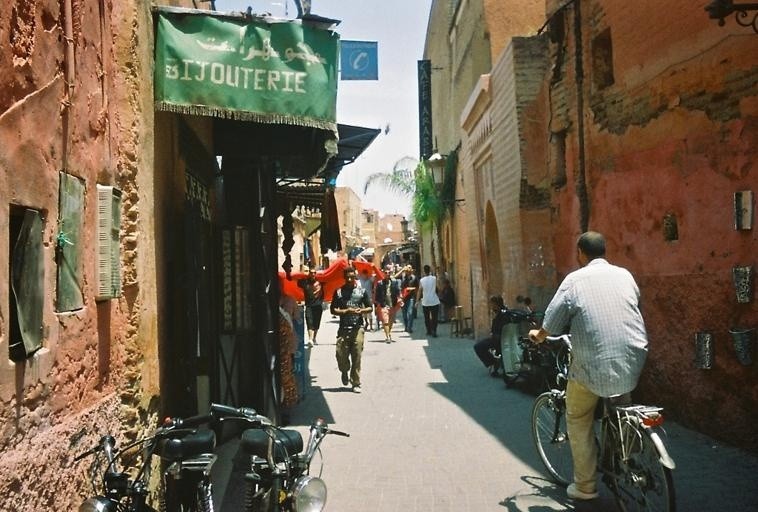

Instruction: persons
[330,268,373,392]
[415,264,442,336]
[358,262,420,343]
[437,279,453,323]
[298,268,324,346]
[474,294,510,374]
[529,230,648,500]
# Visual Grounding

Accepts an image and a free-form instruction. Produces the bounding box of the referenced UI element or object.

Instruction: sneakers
[567,483,600,500]
[387,337,396,343]
[308,340,318,347]
[352,386,361,393]
[342,372,348,385]
[488,363,495,376]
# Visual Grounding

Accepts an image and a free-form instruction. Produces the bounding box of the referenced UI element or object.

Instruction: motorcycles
[74,402,351,511]
[490,295,572,396]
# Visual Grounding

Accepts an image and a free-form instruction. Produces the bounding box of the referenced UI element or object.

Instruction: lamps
[426,148,465,203]
[400,216,423,241]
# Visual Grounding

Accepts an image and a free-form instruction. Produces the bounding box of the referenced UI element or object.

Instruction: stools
[450,317,472,338]
[488,347,502,376]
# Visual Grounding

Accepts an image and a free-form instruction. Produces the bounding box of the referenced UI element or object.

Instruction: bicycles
[532,332,676,511]
